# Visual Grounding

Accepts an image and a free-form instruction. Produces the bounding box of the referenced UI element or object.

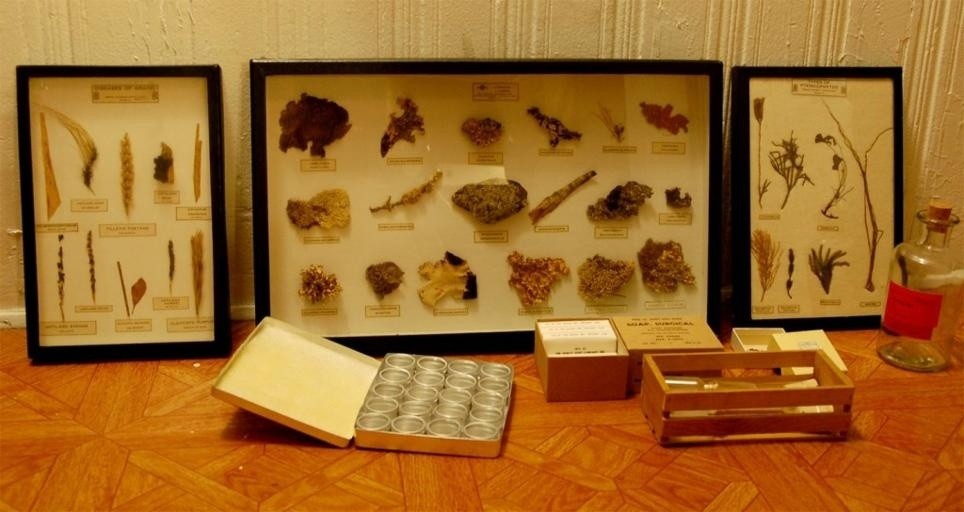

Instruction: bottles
[665,375,834,418]
[875,200,964,373]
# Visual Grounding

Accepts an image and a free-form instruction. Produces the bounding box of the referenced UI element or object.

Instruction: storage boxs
[207,316,516,458]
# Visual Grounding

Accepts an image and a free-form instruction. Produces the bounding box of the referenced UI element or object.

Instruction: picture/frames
[15,57,232,364]
[248,55,725,356]
[731,61,910,337]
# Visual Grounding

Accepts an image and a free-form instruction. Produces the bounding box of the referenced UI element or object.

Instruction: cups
[356,353,511,442]
